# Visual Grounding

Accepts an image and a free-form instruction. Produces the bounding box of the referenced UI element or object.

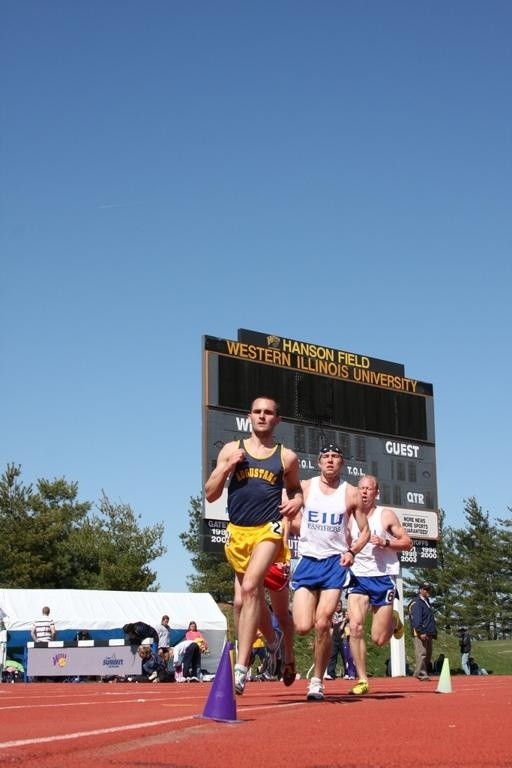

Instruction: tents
[0,588,227,683]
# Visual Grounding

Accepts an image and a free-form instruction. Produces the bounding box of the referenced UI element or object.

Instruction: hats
[419,580,433,588]
[457,627,467,633]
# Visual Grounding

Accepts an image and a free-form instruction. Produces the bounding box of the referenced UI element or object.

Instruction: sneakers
[305,677,324,701]
[282,661,296,687]
[343,674,355,680]
[232,663,247,696]
[348,678,370,695]
[324,675,335,680]
[262,628,283,676]
[391,609,404,639]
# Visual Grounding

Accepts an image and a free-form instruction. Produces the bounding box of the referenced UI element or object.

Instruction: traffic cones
[431,655,456,695]
[192,642,247,727]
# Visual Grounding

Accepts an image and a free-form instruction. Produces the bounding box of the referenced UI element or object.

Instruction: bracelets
[384,539,390,547]
[347,550,354,557]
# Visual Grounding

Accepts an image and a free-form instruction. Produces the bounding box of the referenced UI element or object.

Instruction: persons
[408,584,437,680]
[283,442,371,701]
[124,616,207,682]
[324,601,355,680]
[245,604,293,681]
[433,653,444,675]
[30,606,55,642]
[345,475,413,695]
[457,628,488,676]
[204,394,303,695]
[233,488,303,687]
